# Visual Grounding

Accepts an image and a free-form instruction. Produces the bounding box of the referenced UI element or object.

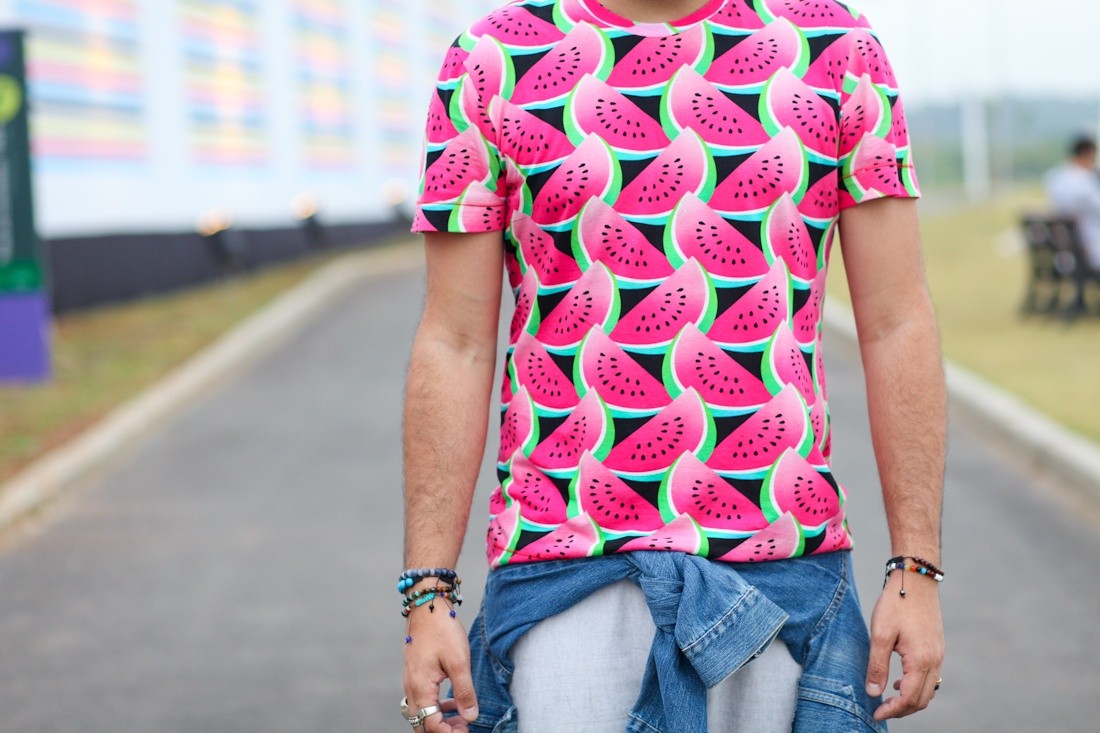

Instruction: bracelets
[883,555,944,600]
[397,567,464,618]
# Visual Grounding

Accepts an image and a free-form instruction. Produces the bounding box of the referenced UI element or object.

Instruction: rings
[407,715,422,728]
[399,697,410,715]
[417,705,440,721]
[936,677,942,689]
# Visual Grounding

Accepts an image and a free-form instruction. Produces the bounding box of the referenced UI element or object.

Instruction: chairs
[1023,216,1100,321]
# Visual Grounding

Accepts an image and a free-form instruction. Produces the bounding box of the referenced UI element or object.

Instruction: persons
[1045,131,1100,274]
[390,1,953,733]
